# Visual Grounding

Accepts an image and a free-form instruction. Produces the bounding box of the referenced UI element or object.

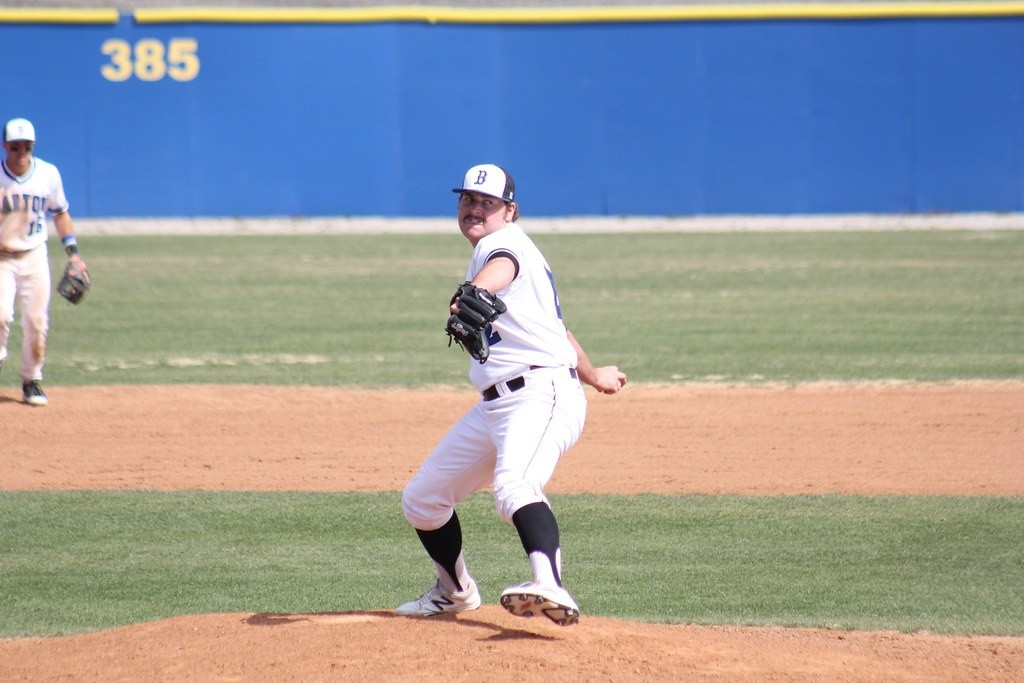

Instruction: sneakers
[499,582,580,626]
[395,579,481,616]
[22,379,48,405]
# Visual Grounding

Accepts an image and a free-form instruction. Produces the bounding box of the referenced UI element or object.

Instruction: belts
[482,376,525,402]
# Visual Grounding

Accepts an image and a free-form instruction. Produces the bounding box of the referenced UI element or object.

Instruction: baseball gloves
[56,260,92,306]
[444,280,508,364]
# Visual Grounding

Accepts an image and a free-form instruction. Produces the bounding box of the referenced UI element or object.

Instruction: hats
[4,117,36,143]
[452,163,515,202]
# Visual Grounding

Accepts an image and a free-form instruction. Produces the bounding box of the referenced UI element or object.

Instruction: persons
[0,119,91,406]
[396,162,627,627]
